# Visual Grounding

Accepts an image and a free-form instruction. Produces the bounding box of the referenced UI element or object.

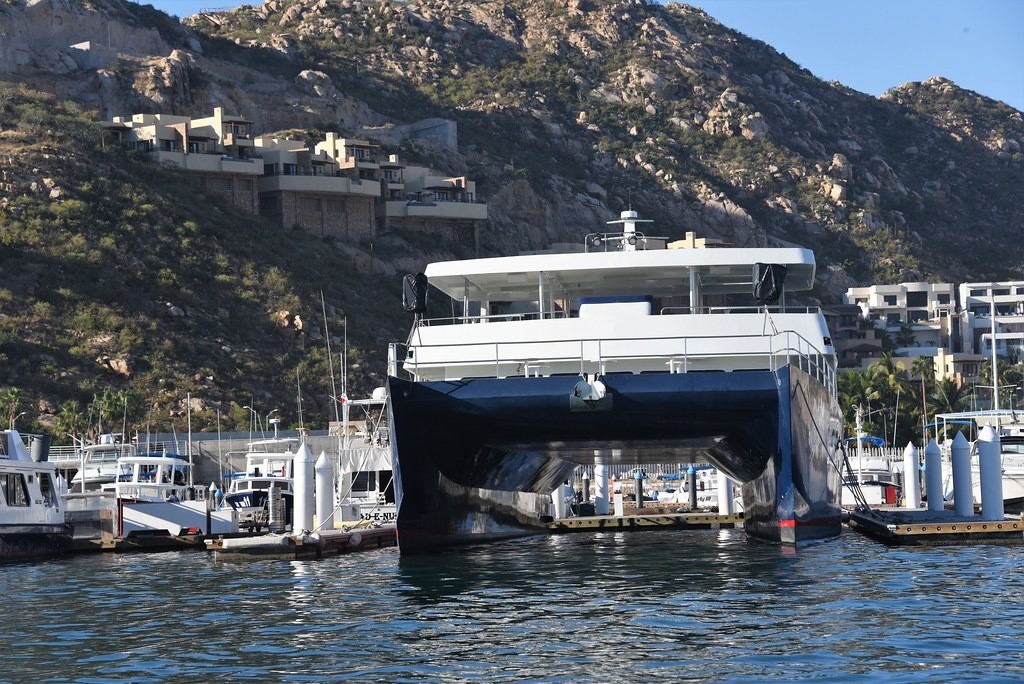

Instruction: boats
[0,287,400,561]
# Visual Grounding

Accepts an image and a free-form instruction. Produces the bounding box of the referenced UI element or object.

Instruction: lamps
[591,232,600,246]
[824,336,832,346]
[628,232,637,245]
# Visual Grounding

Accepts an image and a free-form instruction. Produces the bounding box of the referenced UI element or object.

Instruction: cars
[621,467,653,481]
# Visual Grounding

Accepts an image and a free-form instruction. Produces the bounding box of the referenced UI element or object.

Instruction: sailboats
[656,292,1024,513]
